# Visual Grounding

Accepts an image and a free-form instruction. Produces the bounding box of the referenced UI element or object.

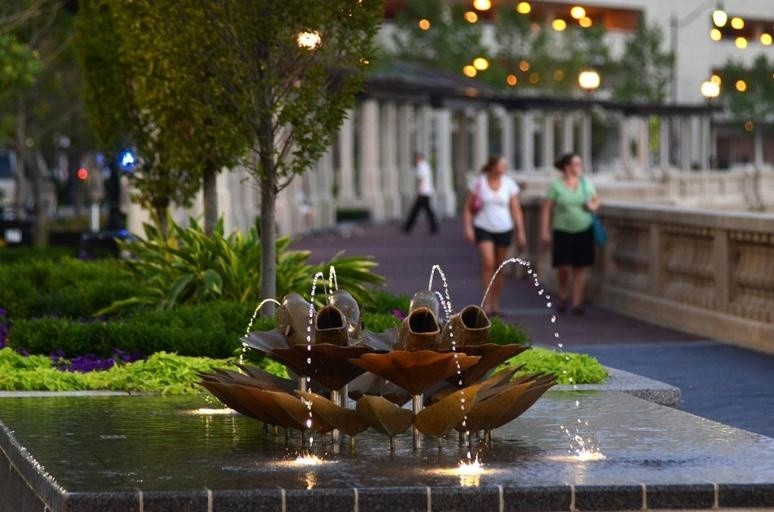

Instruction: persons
[464,154,524,314]
[539,151,602,316]
[399,152,439,236]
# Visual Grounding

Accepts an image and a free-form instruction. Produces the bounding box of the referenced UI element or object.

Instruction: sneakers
[555,299,587,318]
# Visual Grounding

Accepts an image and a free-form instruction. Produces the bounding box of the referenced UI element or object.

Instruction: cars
[0,146,137,246]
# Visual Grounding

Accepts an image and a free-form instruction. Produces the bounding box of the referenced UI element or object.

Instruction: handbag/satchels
[591,211,611,246]
[468,192,483,216]
